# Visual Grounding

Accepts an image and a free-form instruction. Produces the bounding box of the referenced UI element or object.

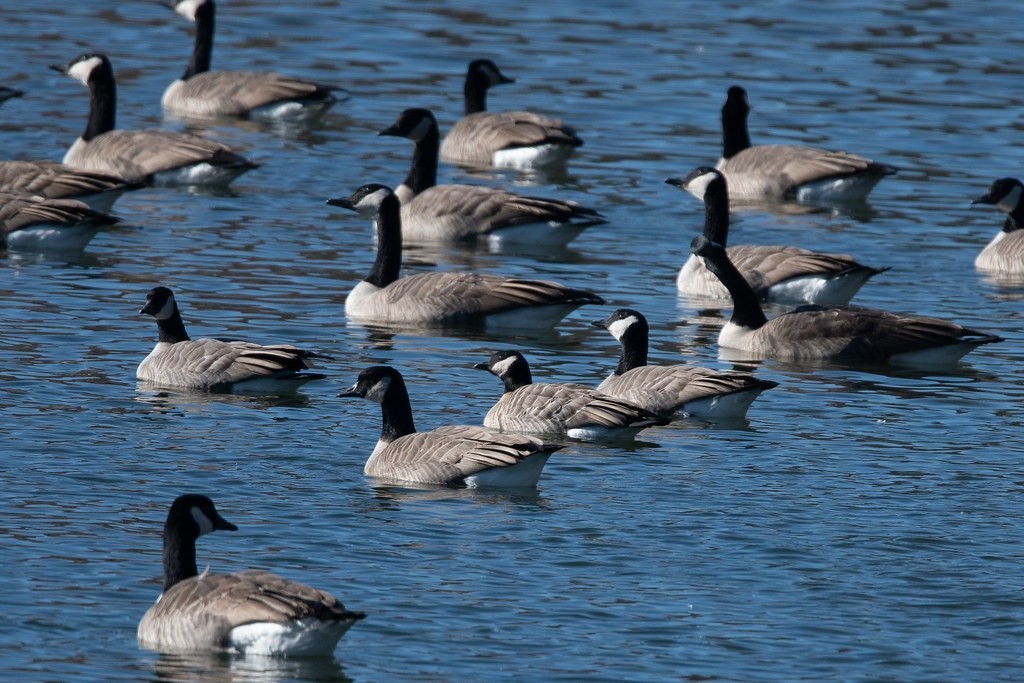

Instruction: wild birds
[138,495,368,656]
[0,0,1024,496]
